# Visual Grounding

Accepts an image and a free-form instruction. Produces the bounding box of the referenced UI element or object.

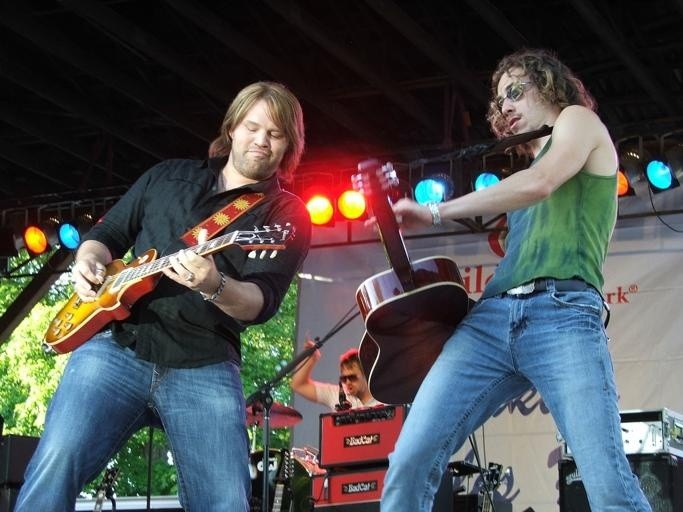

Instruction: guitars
[44,223,296,353]
[350,161,476,405]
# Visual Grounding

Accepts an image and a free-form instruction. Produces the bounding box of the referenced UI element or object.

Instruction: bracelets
[200,271,226,303]
[425,201,442,230]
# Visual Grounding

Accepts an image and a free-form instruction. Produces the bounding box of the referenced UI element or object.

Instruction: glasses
[340,374,357,383]
[495,81,538,108]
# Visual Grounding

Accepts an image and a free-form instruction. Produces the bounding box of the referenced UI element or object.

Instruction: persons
[12,80,314,512]
[363,46,659,511]
[288,332,387,413]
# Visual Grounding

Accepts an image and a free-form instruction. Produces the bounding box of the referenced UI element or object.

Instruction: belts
[505,276,609,329]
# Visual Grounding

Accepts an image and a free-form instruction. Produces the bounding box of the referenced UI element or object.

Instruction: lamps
[305,146,500,235]
[24,199,94,254]
[613,131,682,200]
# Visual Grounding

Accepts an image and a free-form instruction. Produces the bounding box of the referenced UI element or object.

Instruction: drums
[250,449,327,512]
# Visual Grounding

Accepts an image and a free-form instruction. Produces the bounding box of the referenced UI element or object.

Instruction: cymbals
[248,402,302,429]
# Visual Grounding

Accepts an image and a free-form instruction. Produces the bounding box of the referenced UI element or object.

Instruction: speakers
[558,454,683,512]
[0,487,18,512]
[0,434,40,488]
